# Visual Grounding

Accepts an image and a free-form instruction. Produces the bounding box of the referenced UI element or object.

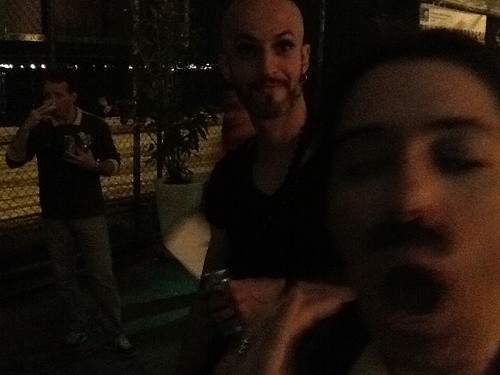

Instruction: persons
[4,61,135,354]
[311,24,499,375]
[173,0,360,374]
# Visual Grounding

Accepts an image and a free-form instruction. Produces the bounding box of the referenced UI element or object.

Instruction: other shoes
[61,332,88,353]
[105,335,136,356]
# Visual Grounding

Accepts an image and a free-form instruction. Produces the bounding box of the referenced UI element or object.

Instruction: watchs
[89,157,101,171]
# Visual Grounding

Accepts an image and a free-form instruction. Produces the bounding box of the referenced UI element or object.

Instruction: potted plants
[130,3,219,254]
